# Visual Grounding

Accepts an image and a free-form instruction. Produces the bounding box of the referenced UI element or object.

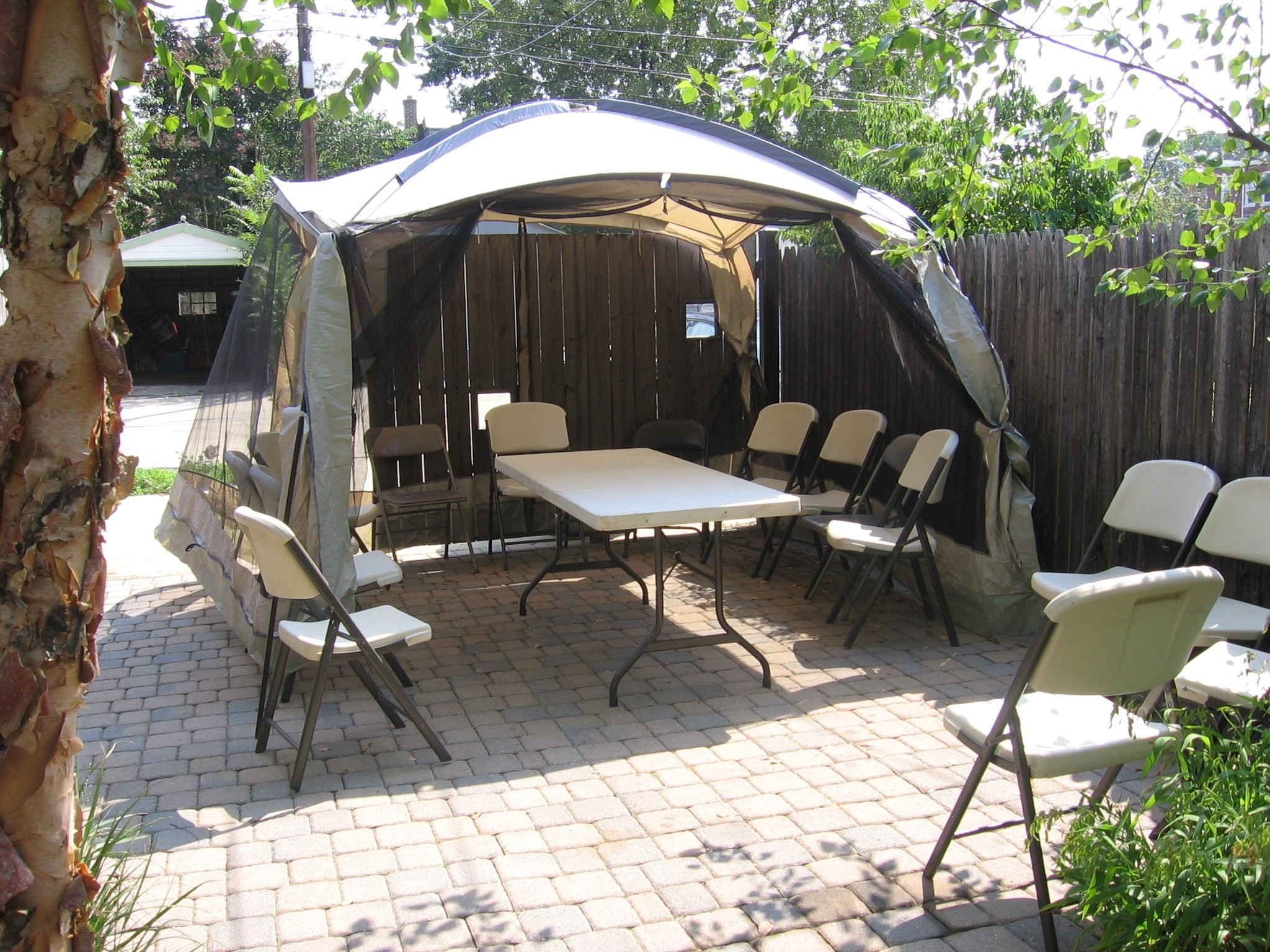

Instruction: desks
[493,443,802,706]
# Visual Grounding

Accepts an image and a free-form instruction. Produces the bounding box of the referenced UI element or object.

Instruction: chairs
[246,431,392,591]
[485,401,591,571]
[225,447,360,553]
[800,432,919,618]
[362,423,482,577]
[1030,458,1222,602]
[621,416,711,559]
[762,408,888,598]
[700,401,822,579]
[1148,639,1270,840]
[247,461,415,702]
[1181,475,1270,648]
[824,427,961,651]
[916,557,1228,952]
[232,502,453,793]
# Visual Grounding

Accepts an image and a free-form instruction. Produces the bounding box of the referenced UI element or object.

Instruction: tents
[153,100,1054,685]
